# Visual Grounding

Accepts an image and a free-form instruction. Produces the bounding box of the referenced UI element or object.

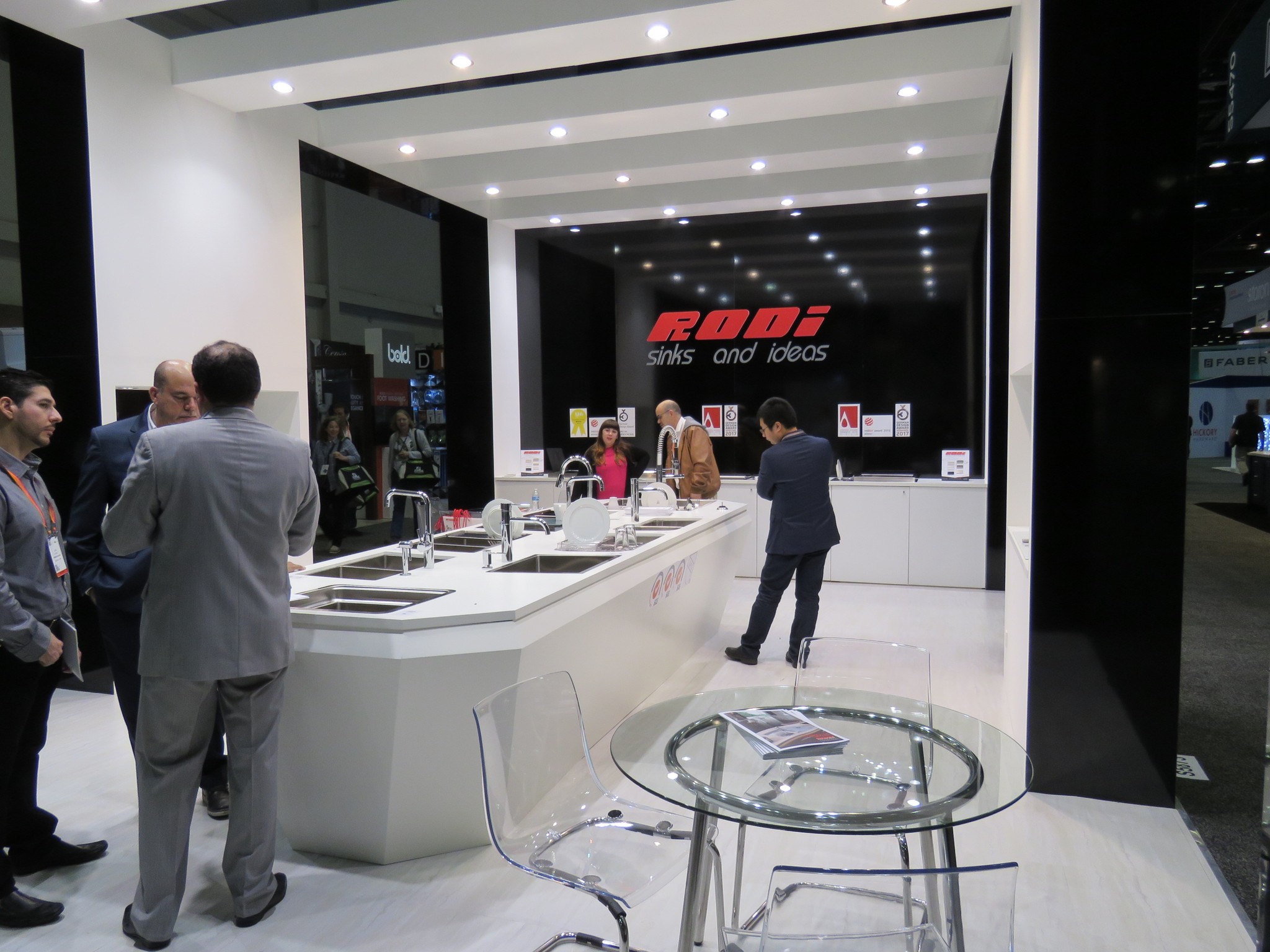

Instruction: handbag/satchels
[392,429,440,491]
[335,437,379,509]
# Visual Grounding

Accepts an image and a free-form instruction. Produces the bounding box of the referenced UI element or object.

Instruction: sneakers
[330,545,341,553]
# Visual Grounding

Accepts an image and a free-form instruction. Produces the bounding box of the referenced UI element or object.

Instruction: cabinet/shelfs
[1247,455,1270,517]
[492,477,987,589]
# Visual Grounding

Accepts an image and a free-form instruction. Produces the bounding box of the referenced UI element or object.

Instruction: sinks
[523,509,556,518]
[293,565,404,581]
[448,530,532,541]
[289,583,458,615]
[486,553,622,575]
[603,498,642,506]
[638,517,702,528]
[342,551,457,573]
[475,514,563,532]
[676,499,717,511]
[614,526,683,532]
[432,535,503,549]
[396,541,488,555]
[604,532,665,544]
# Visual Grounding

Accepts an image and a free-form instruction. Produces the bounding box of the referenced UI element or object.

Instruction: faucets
[383,488,435,568]
[630,478,668,521]
[499,502,551,562]
[655,425,680,484]
[565,475,605,508]
[555,455,593,497]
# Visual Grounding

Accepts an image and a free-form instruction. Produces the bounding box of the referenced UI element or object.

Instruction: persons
[324,401,362,535]
[725,396,840,668]
[1228,399,1266,465]
[388,409,432,542]
[1186,415,1193,459]
[656,400,721,500]
[311,415,360,554]
[0,366,108,929]
[569,419,650,502]
[101,340,320,952]
[66,359,231,819]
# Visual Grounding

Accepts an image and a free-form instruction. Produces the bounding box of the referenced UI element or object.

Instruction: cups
[626,497,631,507]
[615,528,629,550]
[553,503,566,520]
[623,524,637,549]
[608,497,619,509]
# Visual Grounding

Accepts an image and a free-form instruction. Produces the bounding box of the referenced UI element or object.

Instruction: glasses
[657,410,670,420]
[760,423,772,434]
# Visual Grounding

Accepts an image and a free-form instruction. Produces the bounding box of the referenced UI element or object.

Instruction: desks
[609,688,1033,952]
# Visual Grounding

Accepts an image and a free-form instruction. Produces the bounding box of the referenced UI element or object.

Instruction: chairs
[471,636,1020,952]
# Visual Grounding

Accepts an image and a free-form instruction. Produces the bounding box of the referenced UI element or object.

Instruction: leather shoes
[123,902,171,950]
[235,873,287,927]
[725,647,758,665]
[0,891,64,926]
[786,652,806,668]
[202,788,231,817]
[8,838,108,877]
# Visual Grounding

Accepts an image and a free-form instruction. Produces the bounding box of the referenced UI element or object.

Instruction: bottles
[532,489,539,509]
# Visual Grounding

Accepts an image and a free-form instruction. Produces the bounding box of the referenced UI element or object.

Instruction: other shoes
[391,536,400,543]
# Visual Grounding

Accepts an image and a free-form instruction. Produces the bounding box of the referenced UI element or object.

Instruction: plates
[482,499,524,539]
[836,459,843,478]
[641,482,677,508]
[562,498,610,542]
